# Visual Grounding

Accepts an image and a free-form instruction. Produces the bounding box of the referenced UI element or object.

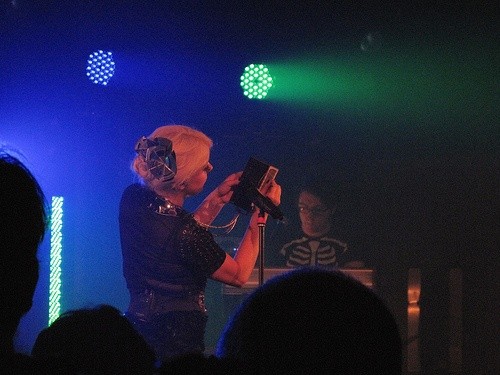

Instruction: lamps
[229,57,274,99]
[84,50,123,90]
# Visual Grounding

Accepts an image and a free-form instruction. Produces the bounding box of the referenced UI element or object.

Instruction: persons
[32,267,404,375]
[0,149,48,375]
[118,124,281,375]
[278,185,355,269]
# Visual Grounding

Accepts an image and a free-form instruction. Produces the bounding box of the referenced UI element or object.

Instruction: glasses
[297,206,328,215]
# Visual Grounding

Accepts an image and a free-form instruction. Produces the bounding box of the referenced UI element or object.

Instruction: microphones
[244,182,284,221]
